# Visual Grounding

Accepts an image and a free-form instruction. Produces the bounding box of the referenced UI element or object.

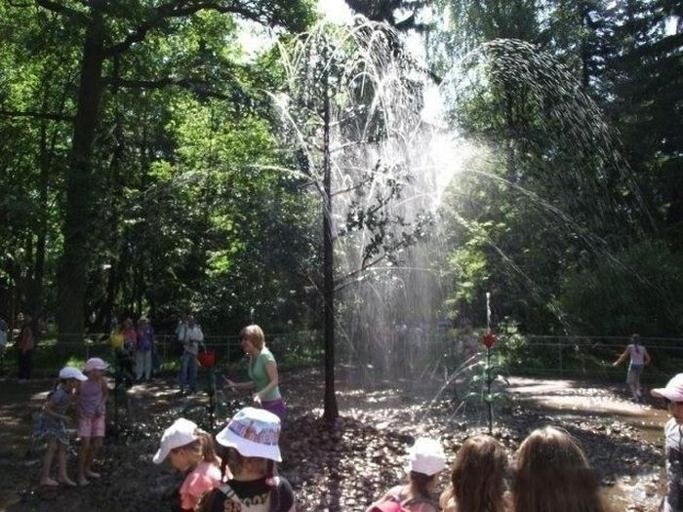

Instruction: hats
[651,373,683,402]
[153,418,197,465]
[137,315,148,323]
[408,436,446,475]
[59,366,88,381]
[86,358,106,371]
[216,406,283,463]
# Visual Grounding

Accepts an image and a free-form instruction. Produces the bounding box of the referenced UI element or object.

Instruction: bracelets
[99,403,102,407]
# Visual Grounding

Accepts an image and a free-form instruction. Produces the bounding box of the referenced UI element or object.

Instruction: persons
[153,417,234,511]
[648,366,683,512]
[1,314,8,358]
[223,324,284,420]
[437,433,513,511]
[611,332,651,403]
[70,357,109,486]
[194,406,295,512]
[39,366,88,487]
[362,437,447,511]
[110,316,156,384]
[173,315,220,396]
[13,316,35,385]
[55,334,66,370]
[350,311,481,401]
[510,422,627,511]
